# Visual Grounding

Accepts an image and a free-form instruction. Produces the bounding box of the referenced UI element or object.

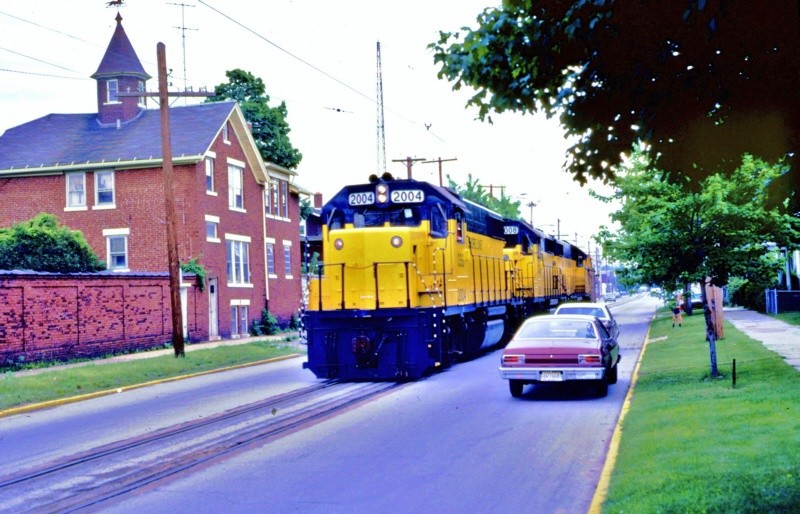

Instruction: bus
[299,173,597,383]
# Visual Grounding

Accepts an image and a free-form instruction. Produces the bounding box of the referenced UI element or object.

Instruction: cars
[603,291,621,301]
[500,301,622,398]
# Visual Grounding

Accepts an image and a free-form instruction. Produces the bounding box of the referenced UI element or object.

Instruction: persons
[672,293,684,328]
